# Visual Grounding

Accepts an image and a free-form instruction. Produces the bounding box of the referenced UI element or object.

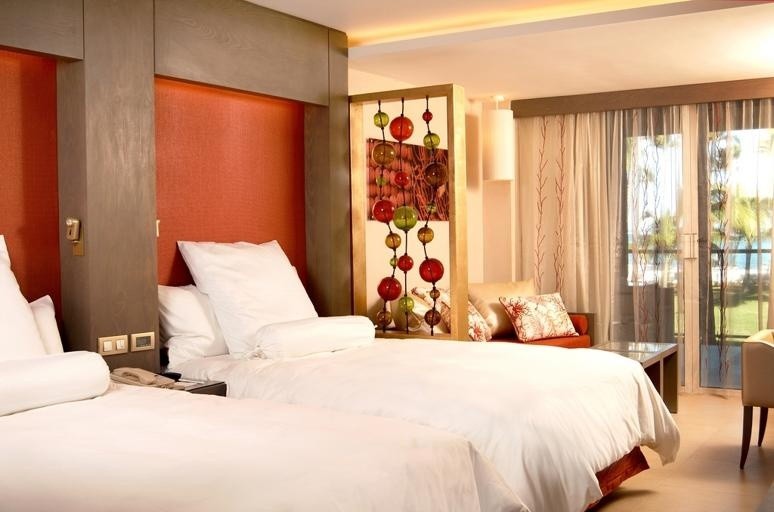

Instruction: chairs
[740,328,774,469]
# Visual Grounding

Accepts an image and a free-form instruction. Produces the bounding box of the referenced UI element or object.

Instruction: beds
[160,337,681,512]
[0,382,532,512]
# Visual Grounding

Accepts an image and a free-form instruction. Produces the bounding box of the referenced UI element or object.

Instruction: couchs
[492,312,598,348]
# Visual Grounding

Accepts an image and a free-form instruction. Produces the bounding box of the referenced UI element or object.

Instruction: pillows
[29,295,64,355]
[250,314,378,361]
[176,238,318,360]
[467,278,538,338]
[0,233,46,360]
[157,282,230,369]
[412,286,493,342]
[569,315,588,334]
[389,290,447,335]
[498,291,580,343]
[0,350,111,418]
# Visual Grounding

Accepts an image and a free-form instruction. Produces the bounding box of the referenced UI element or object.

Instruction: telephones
[110,367,175,389]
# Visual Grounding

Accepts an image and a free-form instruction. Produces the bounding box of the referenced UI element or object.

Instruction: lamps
[480,95,518,183]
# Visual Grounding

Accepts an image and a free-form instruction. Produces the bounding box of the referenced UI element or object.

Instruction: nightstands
[166,377,227,398]
